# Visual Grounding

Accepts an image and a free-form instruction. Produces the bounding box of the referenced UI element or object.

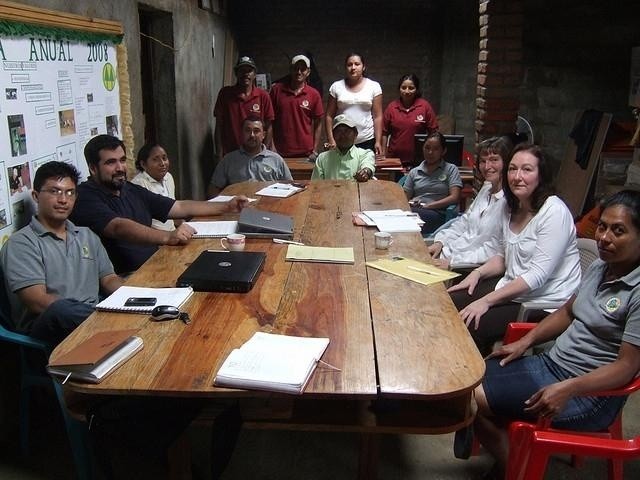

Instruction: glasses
[38,188,77,198]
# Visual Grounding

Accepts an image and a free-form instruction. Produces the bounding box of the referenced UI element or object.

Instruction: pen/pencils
[386,214,417,217]
[273,238,306,246]
[274,187,289,190]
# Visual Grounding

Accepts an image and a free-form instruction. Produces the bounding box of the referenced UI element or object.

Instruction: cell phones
[125,297,157,306]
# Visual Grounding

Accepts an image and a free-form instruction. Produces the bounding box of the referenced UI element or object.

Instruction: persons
[9,167,24,193]
[446,142,582,340]
[382,73,439,165]
[75,134,248,280]
[311,113,377,182]
[1,161,130,351]
[324,51,382,155]
[213,56,276,161]
[130,142,183,234]
[472,189,639,479]
[428,135,513,267]
[11,126,22,156]
[110,121,118,139]
[206,114,294,199]
[269,54,325,162]
[404,132,464,234]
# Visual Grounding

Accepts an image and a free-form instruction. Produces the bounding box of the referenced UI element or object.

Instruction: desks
[49,180,486,476]
[283,158,402,180]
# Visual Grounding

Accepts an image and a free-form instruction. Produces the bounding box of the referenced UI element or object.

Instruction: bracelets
[473,268,483,275]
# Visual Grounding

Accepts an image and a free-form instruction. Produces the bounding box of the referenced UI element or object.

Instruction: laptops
[176,249,265,293]
[238,208,294,239]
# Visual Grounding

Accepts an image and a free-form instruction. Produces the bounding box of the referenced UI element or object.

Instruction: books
[363,208,422,233]
[211,331,330,395]
[95,284,194,316]
[46,328,144,385]
[366,255,461,286]
[255,181,306,198]
[184,217,238,240]
[285,243,353,264]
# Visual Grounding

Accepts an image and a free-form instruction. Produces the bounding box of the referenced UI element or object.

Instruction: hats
[235,56,256,70]
[292,54,310,68]
[332,114,357,130]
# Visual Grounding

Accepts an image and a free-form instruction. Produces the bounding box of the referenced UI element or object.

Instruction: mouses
[152,305,180,321]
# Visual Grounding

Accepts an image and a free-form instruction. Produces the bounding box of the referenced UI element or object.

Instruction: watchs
[363,169,370,176]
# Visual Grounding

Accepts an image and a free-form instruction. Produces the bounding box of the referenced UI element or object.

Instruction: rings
[538,411,546,418]
[466,310,471,314]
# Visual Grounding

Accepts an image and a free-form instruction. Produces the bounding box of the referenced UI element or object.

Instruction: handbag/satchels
[84,394,209,450]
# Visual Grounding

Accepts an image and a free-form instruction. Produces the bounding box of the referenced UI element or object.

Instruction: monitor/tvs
[413,134,464,167]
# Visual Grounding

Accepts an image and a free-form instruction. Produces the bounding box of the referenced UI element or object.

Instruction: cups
[220,233,246,251]
[374,232,394,251]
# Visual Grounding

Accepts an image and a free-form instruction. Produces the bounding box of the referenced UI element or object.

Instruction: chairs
[0,322,71,432]
[515,236,602,322]
[472,322,640,479]
